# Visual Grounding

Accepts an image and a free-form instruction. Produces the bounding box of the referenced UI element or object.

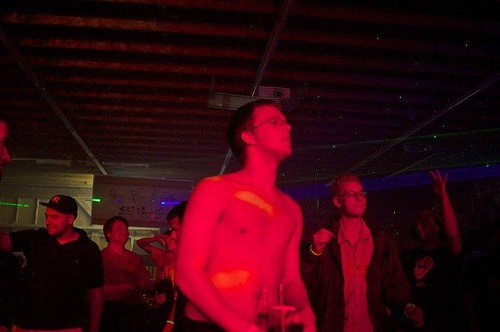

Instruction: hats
[38,195,77,213]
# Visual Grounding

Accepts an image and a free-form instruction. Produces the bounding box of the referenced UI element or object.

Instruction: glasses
[337,191,368,199]
[245,116,288,131]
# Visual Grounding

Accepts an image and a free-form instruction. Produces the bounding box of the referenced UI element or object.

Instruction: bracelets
[166,320,174,324]
[309,243,322,257]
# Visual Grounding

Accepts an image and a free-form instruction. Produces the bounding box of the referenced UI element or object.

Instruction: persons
[401,169,467,332]
[173,100,318,332]
[0,195,104,332]
[100,216,149,332]
[162,199,188,332]
[300,174,425,332]
[0,100,500,332]
[138,230,172,282]
